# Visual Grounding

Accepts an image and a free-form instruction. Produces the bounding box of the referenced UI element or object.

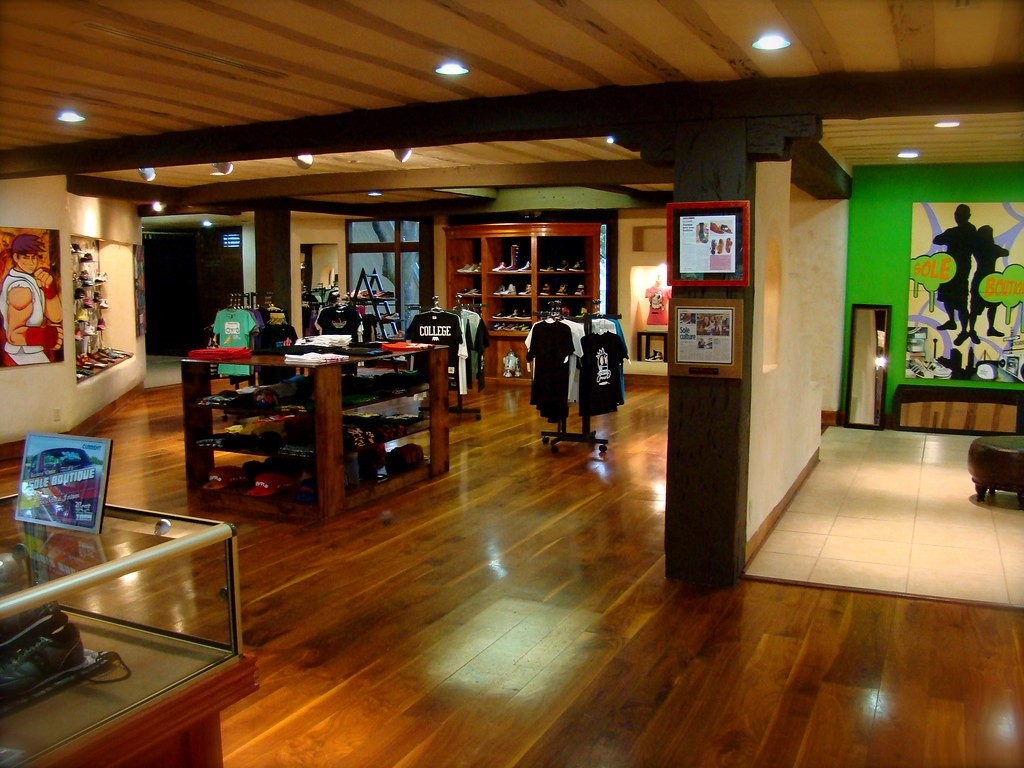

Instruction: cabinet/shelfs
[445,220,602,386]
[179,345,451,522]
[341,268,405,341]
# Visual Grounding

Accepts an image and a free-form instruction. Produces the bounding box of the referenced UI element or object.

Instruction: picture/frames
[17,520,108,580]
[667,298,743,379]
[15,431,113,534]
[666,200,750,287]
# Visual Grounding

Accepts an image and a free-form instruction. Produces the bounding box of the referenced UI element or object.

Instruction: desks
[636,331,668,363]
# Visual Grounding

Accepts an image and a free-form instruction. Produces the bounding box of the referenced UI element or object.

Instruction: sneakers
[72,252,110,341]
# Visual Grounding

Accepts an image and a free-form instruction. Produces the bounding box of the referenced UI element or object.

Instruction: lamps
[391,148,412,163]
[212,162,233,175]
[291,155,313,170]
[139,168,156,182]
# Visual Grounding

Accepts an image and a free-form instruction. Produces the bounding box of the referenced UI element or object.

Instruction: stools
[967,435,1024,511]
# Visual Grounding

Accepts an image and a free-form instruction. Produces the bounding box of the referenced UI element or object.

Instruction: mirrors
[844,304,893,431]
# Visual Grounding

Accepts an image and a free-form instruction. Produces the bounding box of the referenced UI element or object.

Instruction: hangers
[224,293,283,315]
[431,295,444,312]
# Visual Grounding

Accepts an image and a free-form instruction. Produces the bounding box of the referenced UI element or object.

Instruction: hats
[202,465,249,492]
[295,477,318,504]
[248,471,297,497]
